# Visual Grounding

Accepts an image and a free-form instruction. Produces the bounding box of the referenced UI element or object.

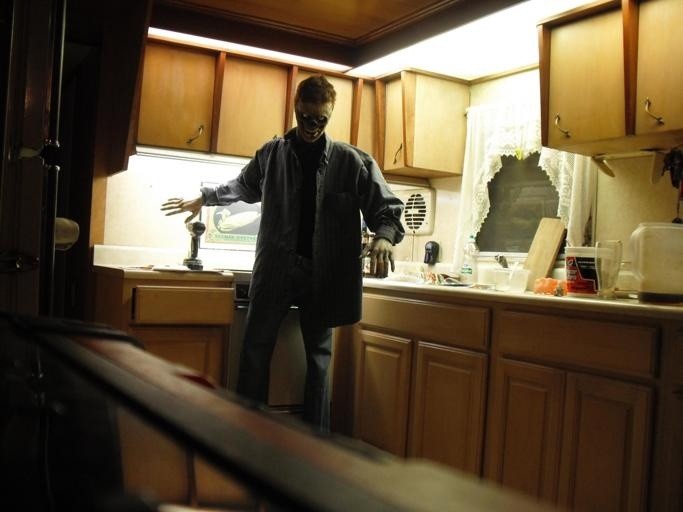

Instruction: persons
[159,75,406,445]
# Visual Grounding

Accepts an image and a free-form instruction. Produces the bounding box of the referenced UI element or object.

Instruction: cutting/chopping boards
[521,218,566,291]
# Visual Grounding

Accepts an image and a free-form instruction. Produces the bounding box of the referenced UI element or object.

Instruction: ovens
[227,273,332,426]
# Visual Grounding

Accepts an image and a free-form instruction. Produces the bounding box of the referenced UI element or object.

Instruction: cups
[629,220,683,304]
[595,240,622,299]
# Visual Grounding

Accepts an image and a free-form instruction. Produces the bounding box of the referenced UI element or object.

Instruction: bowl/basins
[479,266,531,294]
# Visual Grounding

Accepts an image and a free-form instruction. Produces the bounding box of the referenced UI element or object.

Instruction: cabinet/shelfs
[379,72,471,179]
[219,55,375,165]
[485,298,682,507]
[137,41,219,157]
[539,1,683,150]
[347,288,485,473]
[90,269,235,505]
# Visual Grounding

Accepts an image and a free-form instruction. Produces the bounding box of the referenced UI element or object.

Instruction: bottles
[361,231,388,278]
[459,233,479,285]
